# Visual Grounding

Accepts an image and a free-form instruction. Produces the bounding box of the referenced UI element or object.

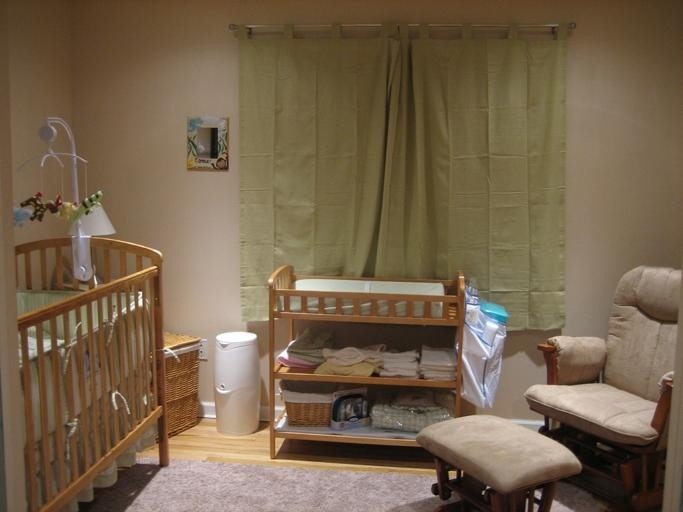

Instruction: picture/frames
[186,114,229,171]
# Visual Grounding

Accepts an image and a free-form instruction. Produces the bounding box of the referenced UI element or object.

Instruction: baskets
[279,380,332,427]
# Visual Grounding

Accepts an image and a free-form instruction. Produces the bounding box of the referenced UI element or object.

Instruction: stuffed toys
[11,191,104,229]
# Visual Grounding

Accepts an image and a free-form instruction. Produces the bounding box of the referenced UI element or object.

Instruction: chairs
[519,262,682,512]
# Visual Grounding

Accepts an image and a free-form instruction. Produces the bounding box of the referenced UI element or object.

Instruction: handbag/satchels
[454,292,506,409]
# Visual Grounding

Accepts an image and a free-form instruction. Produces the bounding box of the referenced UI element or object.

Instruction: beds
[8,230,170,512]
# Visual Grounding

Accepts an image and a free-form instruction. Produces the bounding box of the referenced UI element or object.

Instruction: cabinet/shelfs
[265,262,467,459]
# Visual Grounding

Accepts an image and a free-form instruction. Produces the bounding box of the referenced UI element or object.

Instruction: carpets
[77,452,614,512]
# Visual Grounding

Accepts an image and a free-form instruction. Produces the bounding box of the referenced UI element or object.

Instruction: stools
[414,411,583,511]
